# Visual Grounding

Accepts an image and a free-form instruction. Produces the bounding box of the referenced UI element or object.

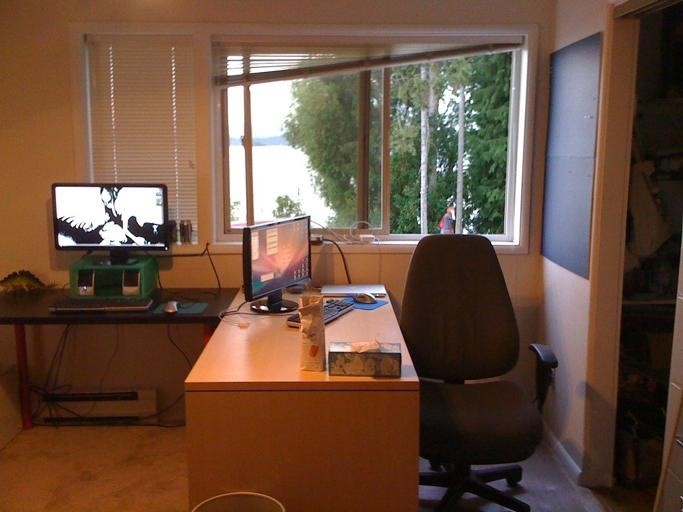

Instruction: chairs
[400,232,562,512]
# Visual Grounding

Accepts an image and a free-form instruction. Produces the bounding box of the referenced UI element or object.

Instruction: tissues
[328,341,402,377]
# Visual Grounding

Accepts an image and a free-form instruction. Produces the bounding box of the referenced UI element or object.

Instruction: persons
[439,206,456,234]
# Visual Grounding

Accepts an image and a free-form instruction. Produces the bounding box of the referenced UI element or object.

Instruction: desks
[181,280,422,511]
[0,285,239,432]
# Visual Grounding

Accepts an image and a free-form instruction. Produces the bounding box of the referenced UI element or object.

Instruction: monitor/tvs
[51,182,169,265]
[243,215,312,313]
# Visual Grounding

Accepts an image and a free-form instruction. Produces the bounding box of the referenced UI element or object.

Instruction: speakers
[77,269,96,296]
[122,269,141,296]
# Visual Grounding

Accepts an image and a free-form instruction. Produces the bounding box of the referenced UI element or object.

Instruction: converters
[310,233,324,241]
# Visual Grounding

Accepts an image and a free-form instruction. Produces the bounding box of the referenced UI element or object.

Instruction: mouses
[164,301,181,313]
[352,293,376,304]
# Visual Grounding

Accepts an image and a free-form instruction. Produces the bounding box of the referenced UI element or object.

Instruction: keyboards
[287,299,354,327]
[49,299,155,312]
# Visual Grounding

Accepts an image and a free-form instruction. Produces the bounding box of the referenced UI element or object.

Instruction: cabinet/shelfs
[618,292,676,483]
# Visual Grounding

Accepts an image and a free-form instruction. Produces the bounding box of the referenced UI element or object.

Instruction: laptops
[320,284,387,297]
